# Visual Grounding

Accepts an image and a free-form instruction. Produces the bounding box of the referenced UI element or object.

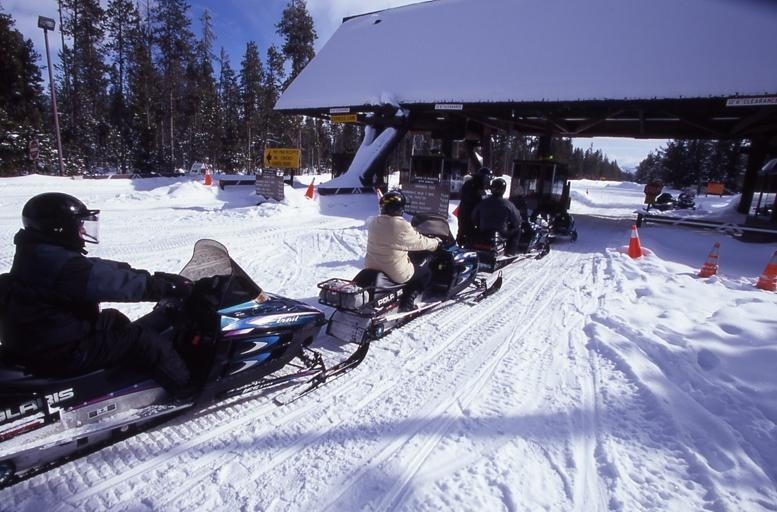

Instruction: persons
[457,167,522,255]
[367,190,442,312]
[0,192,208,403]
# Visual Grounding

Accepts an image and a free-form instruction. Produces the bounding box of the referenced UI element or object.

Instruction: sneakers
[173,372,208,407]
[511,248,522,255]
[403,302,418,311]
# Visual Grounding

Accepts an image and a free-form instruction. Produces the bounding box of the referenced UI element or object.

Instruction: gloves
[152,272,196,312]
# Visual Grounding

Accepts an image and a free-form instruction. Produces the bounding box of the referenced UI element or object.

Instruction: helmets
[380,190,406,215]
[476,167,493,187]
[21,192,99,254]
[491,178,506,194]
